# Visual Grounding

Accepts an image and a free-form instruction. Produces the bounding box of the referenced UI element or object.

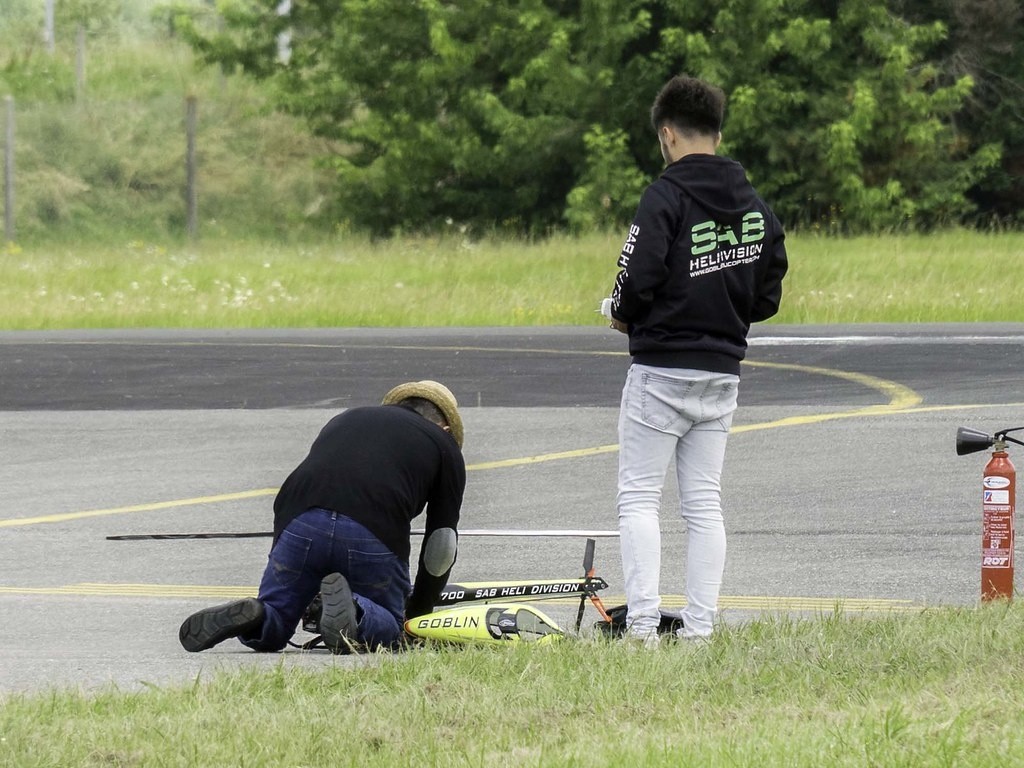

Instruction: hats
[382,379,465,447]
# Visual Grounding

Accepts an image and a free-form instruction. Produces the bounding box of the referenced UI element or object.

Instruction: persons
[179,380,466,655]
[605,75,788,654]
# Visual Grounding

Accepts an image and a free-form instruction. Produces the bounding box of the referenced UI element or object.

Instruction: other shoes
[320,572,359,654]
[179,595,262,652]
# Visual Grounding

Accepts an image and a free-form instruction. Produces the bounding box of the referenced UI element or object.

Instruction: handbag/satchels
[596,603,684,642]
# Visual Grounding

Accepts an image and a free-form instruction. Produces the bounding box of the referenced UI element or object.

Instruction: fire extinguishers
[955,425,1024,605]
